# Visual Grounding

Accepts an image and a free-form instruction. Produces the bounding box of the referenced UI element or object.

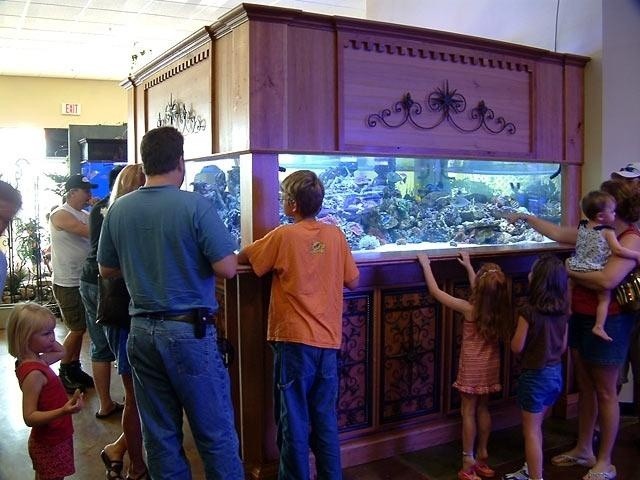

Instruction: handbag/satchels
[613,272,640,305]
[95,272,131,329]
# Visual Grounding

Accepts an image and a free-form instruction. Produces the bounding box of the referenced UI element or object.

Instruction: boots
[58,362,94,394]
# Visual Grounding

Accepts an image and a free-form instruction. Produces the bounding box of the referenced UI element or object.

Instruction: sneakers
[502,461,531,480]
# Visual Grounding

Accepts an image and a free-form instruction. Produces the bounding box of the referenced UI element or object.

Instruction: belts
[136,312,215,324]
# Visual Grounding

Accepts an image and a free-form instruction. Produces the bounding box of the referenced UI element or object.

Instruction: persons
[237,169,362,480]
[8,301,83,480]
[43,127,245,479]
[1,180,23,299]
[415,249,507,480]
[499,162,639,479]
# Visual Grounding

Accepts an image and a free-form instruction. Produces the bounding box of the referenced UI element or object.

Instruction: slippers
[552,451,617,480]
[96,400,124,419]
[458,461,494,480]
[101,449,125,480]
[125,466,149,480]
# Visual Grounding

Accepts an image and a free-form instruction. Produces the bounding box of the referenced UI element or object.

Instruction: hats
[611,163,640,179]
[65,175,98,191]
